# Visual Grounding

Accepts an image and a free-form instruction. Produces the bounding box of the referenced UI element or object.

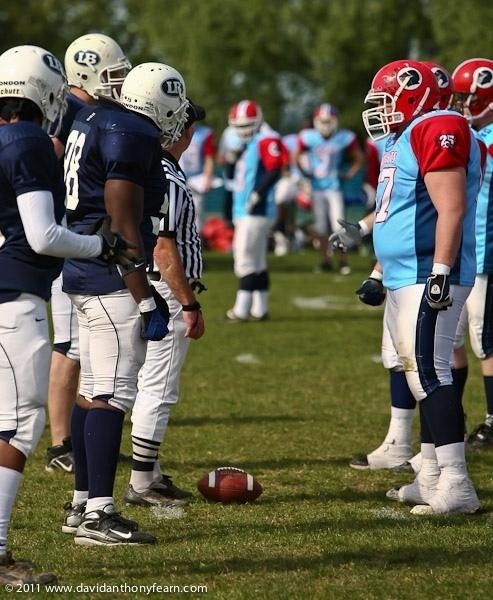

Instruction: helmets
[1,44,72,137]
[228,98,261,142]
[65,33,132,104]
[119,61,189,151]
[362,58,493,142]
[314,103,340,138]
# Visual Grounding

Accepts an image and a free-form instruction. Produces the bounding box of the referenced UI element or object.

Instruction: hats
[185,97,207,126]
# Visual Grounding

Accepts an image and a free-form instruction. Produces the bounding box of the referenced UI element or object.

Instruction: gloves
[140,287,170,341]
[84,214,140,270]
[247,193,266,215]
[424,273,454,312]
[327,218,362,255]
[355,278,386,306]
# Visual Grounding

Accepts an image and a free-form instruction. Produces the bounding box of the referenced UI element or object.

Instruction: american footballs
[197,467,262,503]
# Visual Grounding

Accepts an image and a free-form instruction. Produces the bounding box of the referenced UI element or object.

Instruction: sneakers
[465,417,493,452]
[124,472,192,507]
[222,307,270,323]
[350,446,414,470]
[74,504,157,547]
[315,258,351,275]
[61,501,139,534]
[388,470,480,516]
[46,436,77,473]
[0,552,57,591]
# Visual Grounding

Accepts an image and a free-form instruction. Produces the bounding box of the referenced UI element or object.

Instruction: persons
[441,56,492,448]
[349,57,484,523]
[46,29,130,477]
[0,41,140,588]
[176,100,389,327]
[59,56,190,553]
[121,98,208,513]
[327,61,455,473]
